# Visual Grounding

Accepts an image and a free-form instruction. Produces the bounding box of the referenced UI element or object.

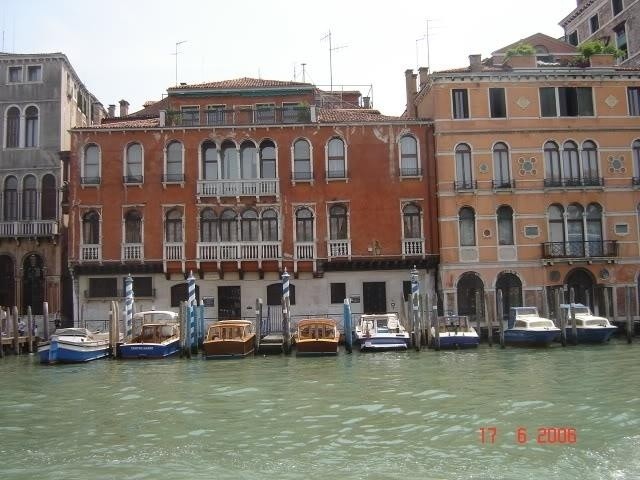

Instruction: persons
[53,316,61,330]
[18,318,27,337]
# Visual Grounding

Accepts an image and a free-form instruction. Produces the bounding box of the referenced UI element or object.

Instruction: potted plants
[580,39,627,68]
[499,44,539,68]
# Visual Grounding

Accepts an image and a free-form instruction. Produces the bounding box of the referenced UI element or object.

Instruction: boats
[354,313,411,352]
[559,303,619,344]
[293,319,341,356]
[36,327,124,364]
[431,315,480,348]
[202,320,257,358]
[495,306,562,346]
[118,310,181,359]
